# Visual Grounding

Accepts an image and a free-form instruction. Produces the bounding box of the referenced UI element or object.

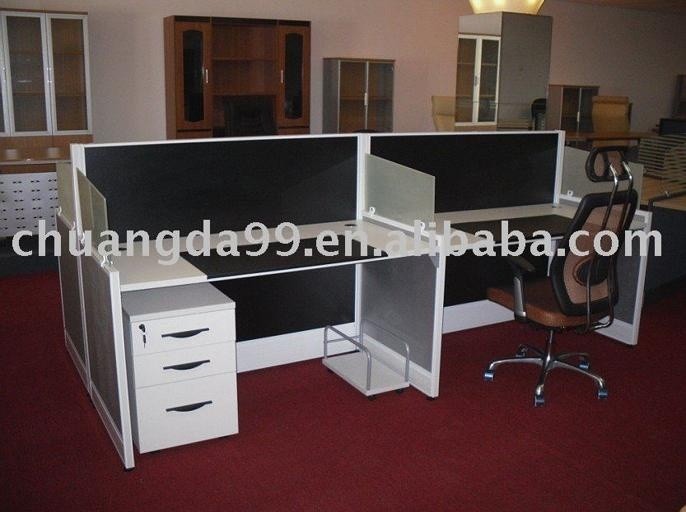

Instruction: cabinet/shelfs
[454,11,554,131]
[545,84,601,147]
[0,7,94,239]
[163,15,311,140]
[322,57,396,134]
